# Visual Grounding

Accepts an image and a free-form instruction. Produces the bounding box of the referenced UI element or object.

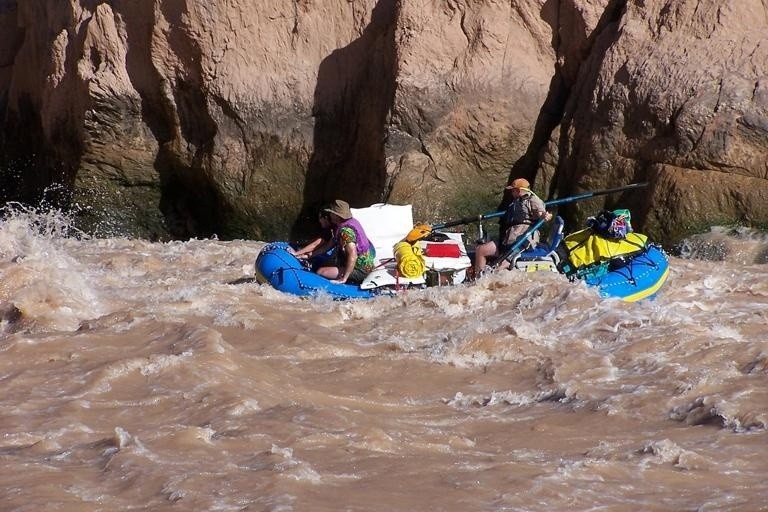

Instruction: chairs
[509,216,564,270]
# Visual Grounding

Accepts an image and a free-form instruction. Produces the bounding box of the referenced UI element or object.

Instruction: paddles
[405,183,647,242]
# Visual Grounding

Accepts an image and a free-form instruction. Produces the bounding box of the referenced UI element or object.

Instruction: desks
[414,232,472,286]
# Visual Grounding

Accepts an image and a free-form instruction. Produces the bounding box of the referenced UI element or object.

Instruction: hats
[324,200,352,219]
[505,179,530,190]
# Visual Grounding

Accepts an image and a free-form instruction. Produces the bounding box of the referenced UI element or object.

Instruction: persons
[473,177,553,278]
[287,206,336,256]
[295,199,375,285]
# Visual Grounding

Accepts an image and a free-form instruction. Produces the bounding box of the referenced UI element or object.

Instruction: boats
[255,242,671,303]
